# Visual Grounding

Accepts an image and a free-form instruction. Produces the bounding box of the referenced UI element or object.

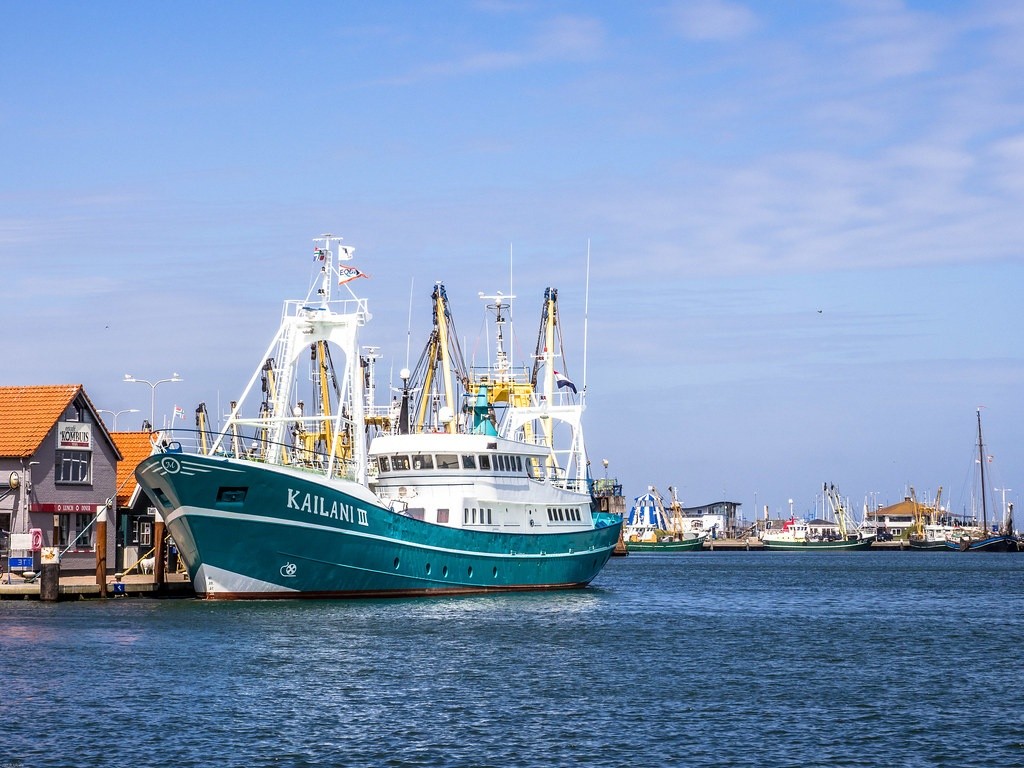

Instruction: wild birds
[816,308,823,316]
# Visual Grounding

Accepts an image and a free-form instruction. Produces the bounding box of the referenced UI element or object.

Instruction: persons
[747,538,749,546]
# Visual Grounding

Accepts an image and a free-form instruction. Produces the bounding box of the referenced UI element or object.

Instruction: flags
[338,246,355,261]
[313,246,325,262]
[174,404,186,420]
[553,370,577,394]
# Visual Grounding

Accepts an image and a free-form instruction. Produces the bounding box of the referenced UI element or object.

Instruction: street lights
[96,408,141,430]
[122,374,184,431]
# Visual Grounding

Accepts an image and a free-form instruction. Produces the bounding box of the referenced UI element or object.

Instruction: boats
[133,234,625,603]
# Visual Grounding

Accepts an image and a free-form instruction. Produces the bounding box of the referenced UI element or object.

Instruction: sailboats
[619,407,1024,552]
